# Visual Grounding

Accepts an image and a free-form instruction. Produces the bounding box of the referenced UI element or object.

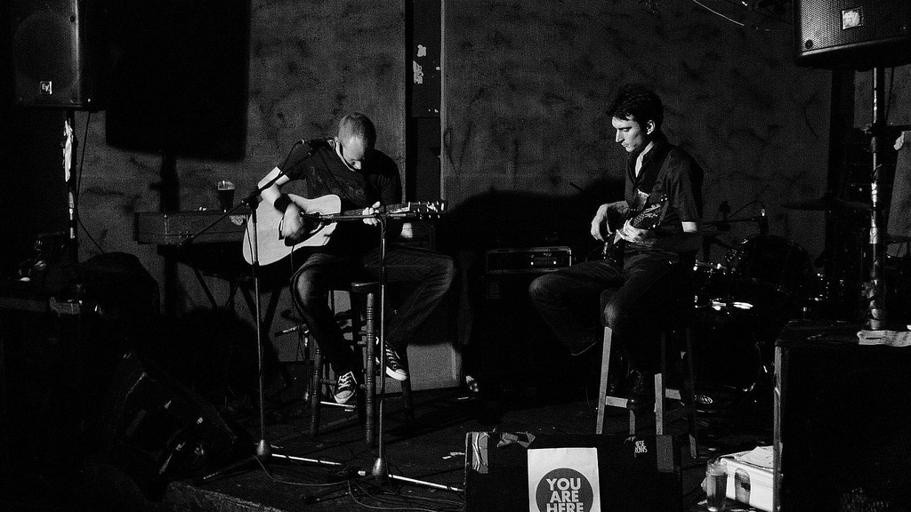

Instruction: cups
[216,188,235,213]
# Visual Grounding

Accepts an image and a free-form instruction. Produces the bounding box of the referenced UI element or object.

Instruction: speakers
[464,432,629,512]
[793,0,911,71]
[9,0,84,109]
[321,289,462,398]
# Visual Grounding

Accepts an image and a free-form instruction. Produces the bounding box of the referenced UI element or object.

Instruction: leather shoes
[593,372,659,413]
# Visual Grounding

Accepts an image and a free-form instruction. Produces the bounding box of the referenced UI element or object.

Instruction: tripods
[181,149,343,481]
[303,207,465,506]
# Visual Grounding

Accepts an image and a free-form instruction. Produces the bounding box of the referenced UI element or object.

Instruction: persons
[528,83,708,419]
[254,110,458,412]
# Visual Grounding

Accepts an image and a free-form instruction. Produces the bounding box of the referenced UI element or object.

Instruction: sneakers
[371,333,408,381]
[334,370,359,403]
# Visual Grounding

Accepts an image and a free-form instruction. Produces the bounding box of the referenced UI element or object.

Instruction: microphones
[299,138,336,150]
[759,207,769,237]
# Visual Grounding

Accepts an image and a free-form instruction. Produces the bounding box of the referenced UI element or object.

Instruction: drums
[736,230,814,299]
[798,266,840,312]
[696,286,802,401]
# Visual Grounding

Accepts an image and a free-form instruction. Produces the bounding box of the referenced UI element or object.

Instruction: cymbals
[786,197,874,211]
[691,217,764,226]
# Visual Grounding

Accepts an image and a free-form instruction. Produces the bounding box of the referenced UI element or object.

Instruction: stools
[595,289,700,470]
[310,279,415,450]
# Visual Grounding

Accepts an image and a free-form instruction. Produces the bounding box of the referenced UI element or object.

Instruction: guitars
[243,193,447,267]
[603,203,661,259]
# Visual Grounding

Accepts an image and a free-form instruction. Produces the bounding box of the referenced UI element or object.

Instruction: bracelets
[273,194,294,213]
